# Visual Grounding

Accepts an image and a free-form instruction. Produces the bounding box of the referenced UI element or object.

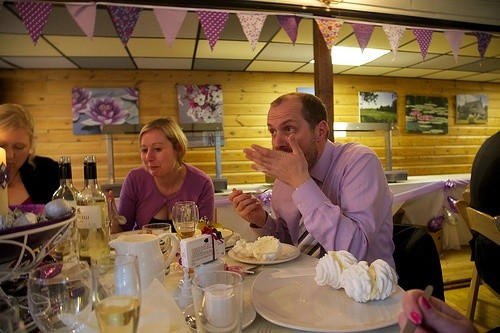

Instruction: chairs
[394,223,446,304]
[453,198,500,319]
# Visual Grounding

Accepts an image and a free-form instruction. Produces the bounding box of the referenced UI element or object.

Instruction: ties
[296,176,321,260]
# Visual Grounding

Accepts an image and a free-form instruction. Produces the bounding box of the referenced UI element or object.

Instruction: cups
[27,260,93,332]
[142,223,171,275]
[190,270,243,333]
[92,253,141,333]
[172,201,199,238]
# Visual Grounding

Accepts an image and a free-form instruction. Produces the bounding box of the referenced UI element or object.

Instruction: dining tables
[88,252,401,333]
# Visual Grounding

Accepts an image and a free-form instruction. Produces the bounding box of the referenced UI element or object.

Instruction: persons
[229,92,397,271]
[468,130,500,296]
[104,117,216,233]
[397,290,479,333]
[0,104,74,213]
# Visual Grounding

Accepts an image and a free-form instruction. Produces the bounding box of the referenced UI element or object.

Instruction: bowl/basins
[216,226,234,245]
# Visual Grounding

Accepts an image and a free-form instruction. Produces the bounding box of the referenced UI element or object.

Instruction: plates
[182,297,257,332]
[228,243,301,264]
[250,267,406,332]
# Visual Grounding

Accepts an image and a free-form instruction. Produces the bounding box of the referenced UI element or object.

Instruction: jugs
[108,232,178,300]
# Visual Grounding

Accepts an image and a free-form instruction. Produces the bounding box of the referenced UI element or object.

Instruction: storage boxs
[180,231,226,267]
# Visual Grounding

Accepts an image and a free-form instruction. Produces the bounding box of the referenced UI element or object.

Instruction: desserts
[233,234,283,262]
[315,248,399,304]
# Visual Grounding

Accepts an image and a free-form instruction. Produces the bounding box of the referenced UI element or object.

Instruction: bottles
[52,156,79,261]
[75,155,110,273]
